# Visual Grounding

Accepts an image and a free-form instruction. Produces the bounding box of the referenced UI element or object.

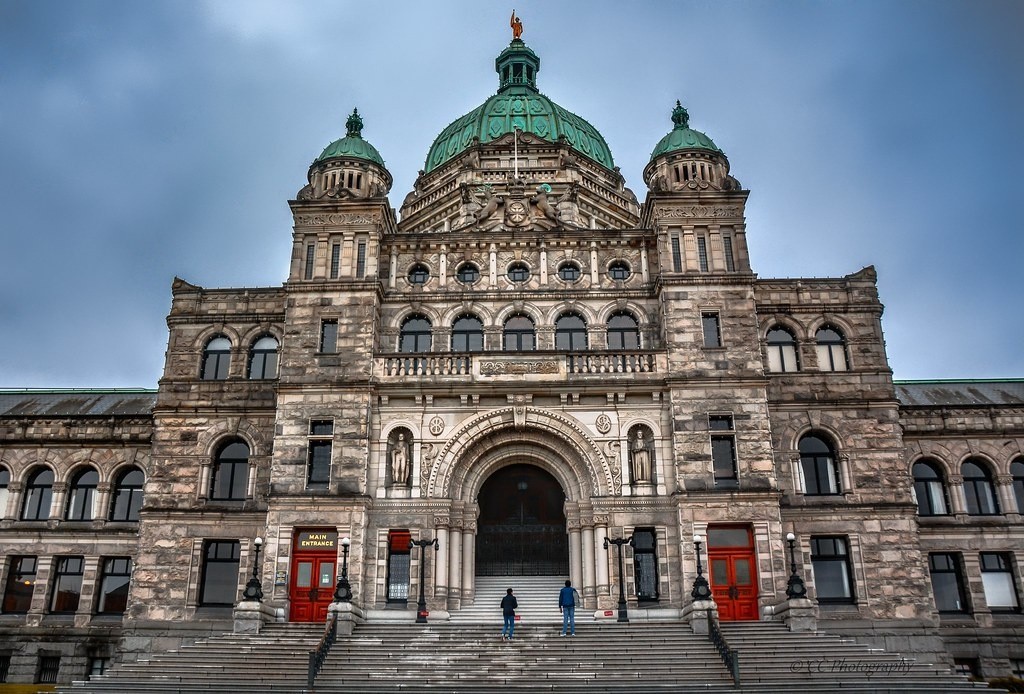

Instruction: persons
[631,430,652,481]
[391,433,409,483]
[558,579,580,637]
[500,588,519,642]
[510,9,523,39]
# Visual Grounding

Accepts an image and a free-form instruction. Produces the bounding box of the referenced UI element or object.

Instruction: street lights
[603,536,636,622]
[406,538,439,624]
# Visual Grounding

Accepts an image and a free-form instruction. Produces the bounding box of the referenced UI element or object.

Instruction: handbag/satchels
[572,591,581,607]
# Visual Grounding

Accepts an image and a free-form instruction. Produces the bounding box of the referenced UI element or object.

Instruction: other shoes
[560,634,566,637]
[508,636,513,640]
[501,634,505,642]
[572,634,575,637]
